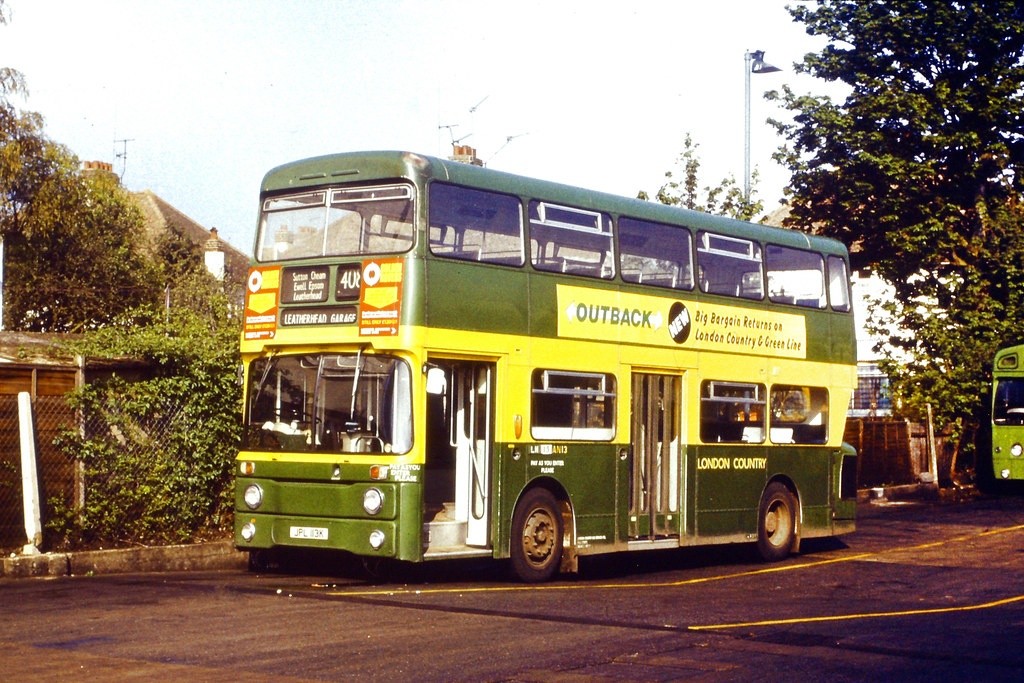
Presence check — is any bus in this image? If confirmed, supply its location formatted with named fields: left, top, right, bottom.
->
left=234, top=149, right=858, bottom=586
left=990, top=345, right=1024, bottom=481
